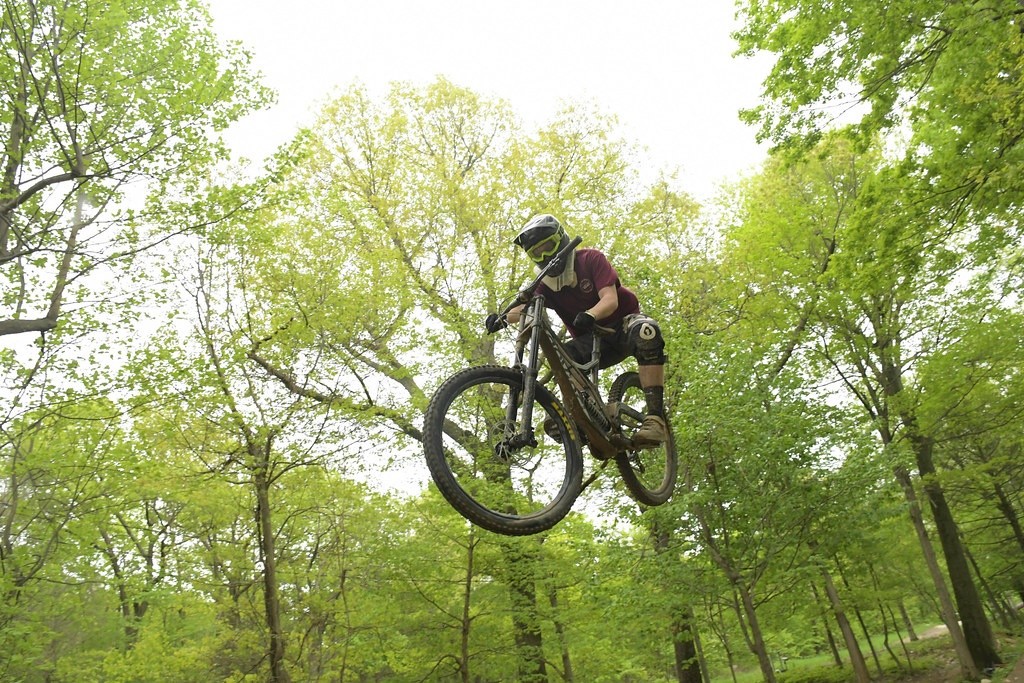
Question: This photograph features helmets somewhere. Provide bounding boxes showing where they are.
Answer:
[514,214,570,277]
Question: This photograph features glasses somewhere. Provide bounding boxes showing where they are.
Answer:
[526,222,564,262]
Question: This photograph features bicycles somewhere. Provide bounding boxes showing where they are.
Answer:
[418,233,681,540]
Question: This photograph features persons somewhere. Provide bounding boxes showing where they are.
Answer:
[487,215,672,445]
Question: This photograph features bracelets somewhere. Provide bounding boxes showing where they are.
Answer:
[586,310,596,321]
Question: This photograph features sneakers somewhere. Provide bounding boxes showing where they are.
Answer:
[544,415,563,444]
[634,415,668,441]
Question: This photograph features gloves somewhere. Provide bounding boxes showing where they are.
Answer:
[486,314,508,333]
[573,312,595,331]
